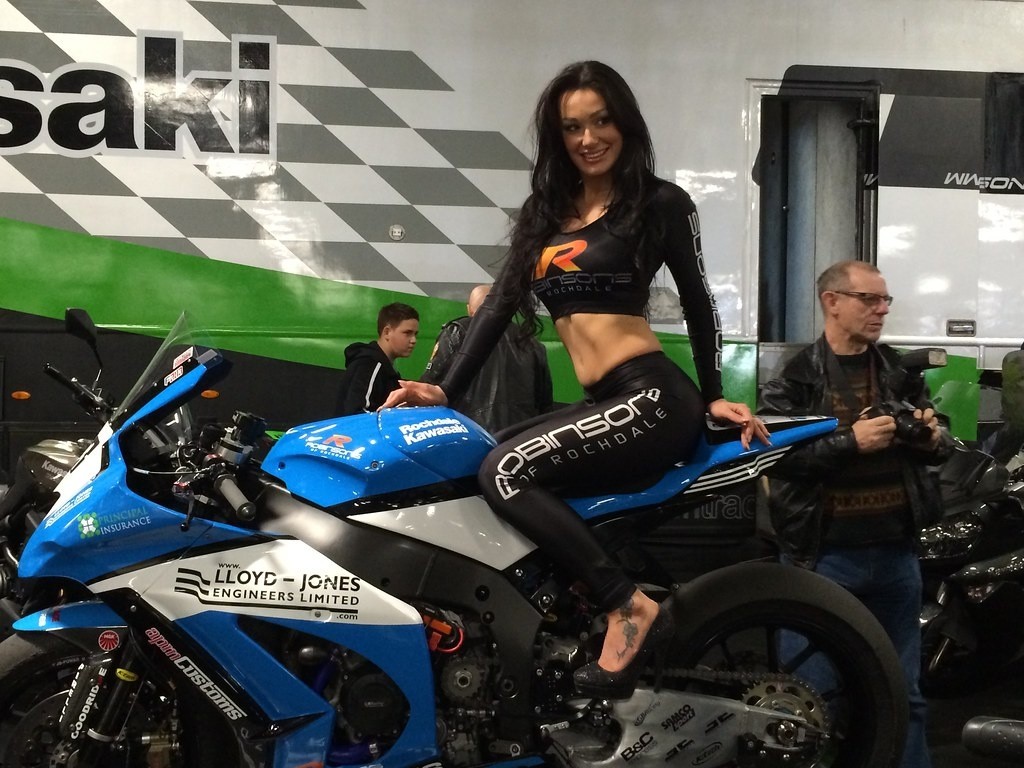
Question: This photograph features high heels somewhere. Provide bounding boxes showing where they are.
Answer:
[573,603,673,698]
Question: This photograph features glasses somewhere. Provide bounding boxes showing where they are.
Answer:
[833,289,893,306]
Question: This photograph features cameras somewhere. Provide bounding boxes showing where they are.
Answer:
[866,399,934,445]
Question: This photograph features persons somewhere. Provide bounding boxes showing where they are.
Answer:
[418,285,555,435]
[377,59,772,698]
[757,262,948,767]
[337,302,421,417]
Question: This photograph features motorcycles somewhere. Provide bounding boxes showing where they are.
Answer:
[917,427,1023,691]
[0,308,909,768]
[962,718,1024,761]
